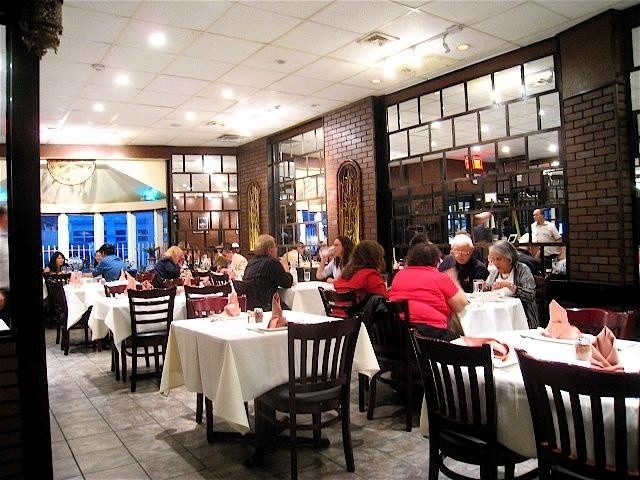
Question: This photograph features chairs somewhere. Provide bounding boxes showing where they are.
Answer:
[565,306,633,341]
[515,346,640,480]
[45,260,519,442]
[408,327,538,480]
[254,316,362,480]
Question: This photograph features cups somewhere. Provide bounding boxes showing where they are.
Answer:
[201,267,205,271]
[472,279,484,294]
[254,308,264,323]
[575,337,592,367]
[61,265,72,273]
[323,248,335,258]
[303,268,310,283]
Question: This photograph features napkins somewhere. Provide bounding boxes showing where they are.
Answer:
[540,299,583,340]
[589,326,625,375]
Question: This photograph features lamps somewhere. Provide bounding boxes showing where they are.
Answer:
[376,29,452,82]
[464,153,484,179]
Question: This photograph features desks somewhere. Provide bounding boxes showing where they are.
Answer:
[428,327,640,476]
[169,310,345,470]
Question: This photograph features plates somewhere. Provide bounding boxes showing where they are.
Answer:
[257,322,288,331]
[491,345,521,368]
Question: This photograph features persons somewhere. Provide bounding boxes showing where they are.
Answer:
[0,205,10,333]
[283,241,305,263]
[153,246,183,288]
[44,251,70,274]
[388,242,467,340]
[531,208,566,269]
[93,244,125,282]
[473,212,499,261]
[206,243,248,281]
[240,235,293,310]
[515,232,540,276]
[316,236,354,283]
[484,239,539,327]
[437,233,490,293]
[332,239,386,317]
